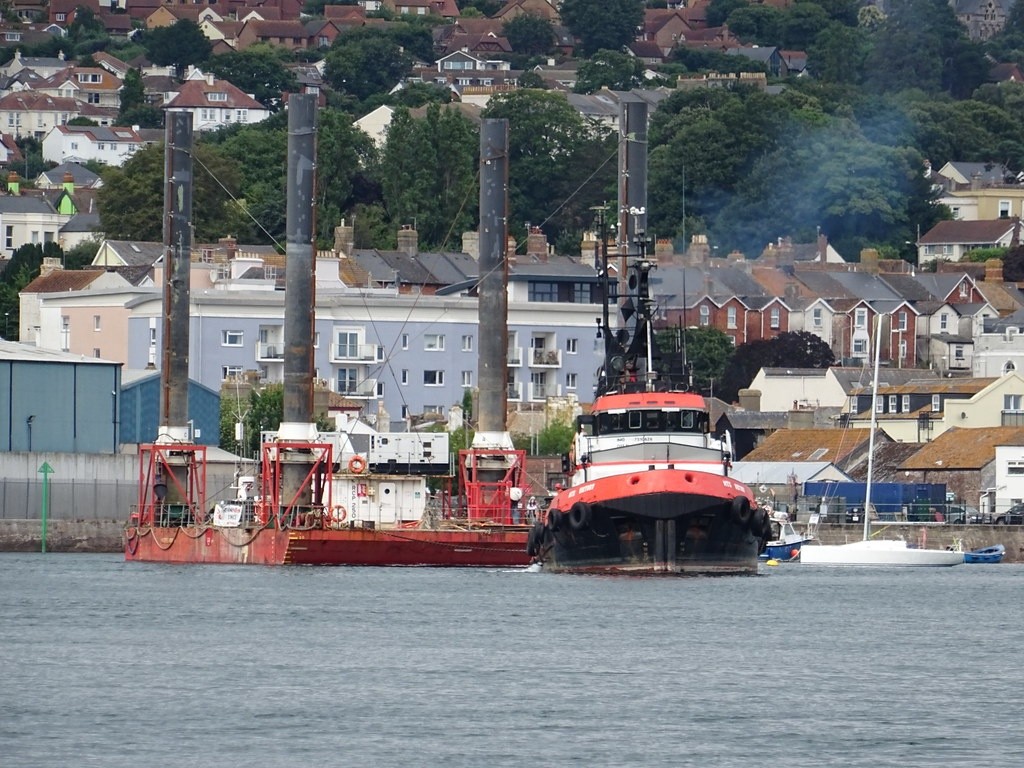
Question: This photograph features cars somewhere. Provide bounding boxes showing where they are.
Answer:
[995,503,1023,530]
[945,491,960,502]
[946,502,982,524]
[843,506,865,524]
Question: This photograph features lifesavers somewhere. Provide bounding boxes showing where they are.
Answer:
[329,506,347,522]
[348,456,366,473]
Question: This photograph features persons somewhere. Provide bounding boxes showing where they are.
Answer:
[554,483,562,494]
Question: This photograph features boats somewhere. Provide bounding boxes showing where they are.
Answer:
[123,89,542,572]
[527,203,780,580]
[758,500,816,564]
[964,542,1007,567]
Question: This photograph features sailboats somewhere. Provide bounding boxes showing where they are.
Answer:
[798,311,967,568]
